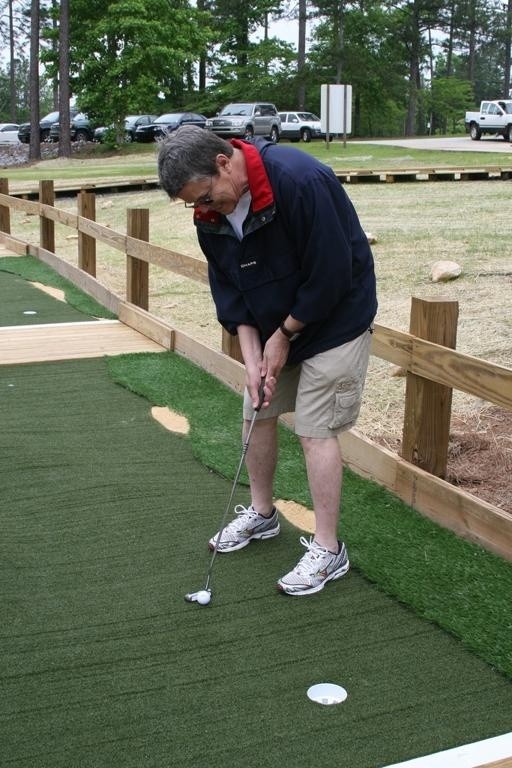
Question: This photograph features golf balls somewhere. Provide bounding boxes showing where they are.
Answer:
[196,590,211,605]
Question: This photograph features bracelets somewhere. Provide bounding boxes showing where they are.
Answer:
[279,319,300,342]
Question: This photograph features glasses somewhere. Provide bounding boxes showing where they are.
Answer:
[185,175,214,209]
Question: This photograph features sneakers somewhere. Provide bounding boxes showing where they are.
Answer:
[208,505,280,553]
[277,536,350,596]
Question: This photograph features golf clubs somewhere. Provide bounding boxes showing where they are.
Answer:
[184,371,266,602]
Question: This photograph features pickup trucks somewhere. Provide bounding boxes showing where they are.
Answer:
[463,99,512,143]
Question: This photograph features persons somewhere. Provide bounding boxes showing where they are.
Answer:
[155,121,377,599]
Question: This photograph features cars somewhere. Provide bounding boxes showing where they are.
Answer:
[0,123,22,147]
[278,111,333,143]
[136,113,208,143]
[93,114,159,144]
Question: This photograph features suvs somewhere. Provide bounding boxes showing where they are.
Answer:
[18,110,78,144]
[49,111,99,143]
[204,101,282,144]
